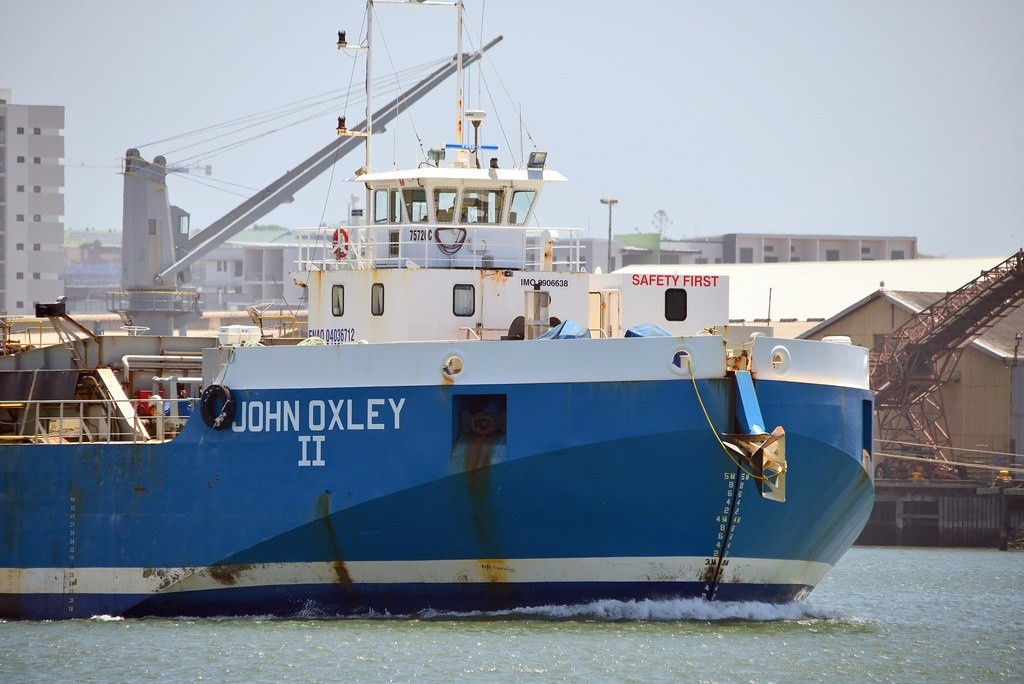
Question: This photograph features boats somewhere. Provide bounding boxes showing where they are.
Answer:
[0,2,878,623]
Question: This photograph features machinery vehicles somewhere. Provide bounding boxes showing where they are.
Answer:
[867,248,1024,480]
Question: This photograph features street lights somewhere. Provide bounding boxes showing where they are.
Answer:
[600,197,619,274]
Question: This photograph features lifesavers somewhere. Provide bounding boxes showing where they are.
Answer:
[333,227,350,258]
[201,382,235,431]
